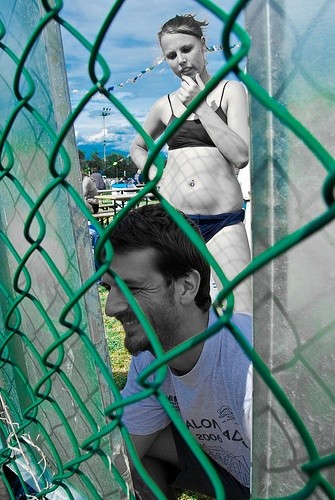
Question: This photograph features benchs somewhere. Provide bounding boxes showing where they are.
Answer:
[93,203,117,225]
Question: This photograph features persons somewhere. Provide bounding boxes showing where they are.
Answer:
[97,203,253,500]
[81,168,142,214]
[131,14,252,315]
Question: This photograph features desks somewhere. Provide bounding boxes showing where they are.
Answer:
[94,184,153,212]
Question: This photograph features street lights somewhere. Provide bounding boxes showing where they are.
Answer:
[102,106,111,164]
